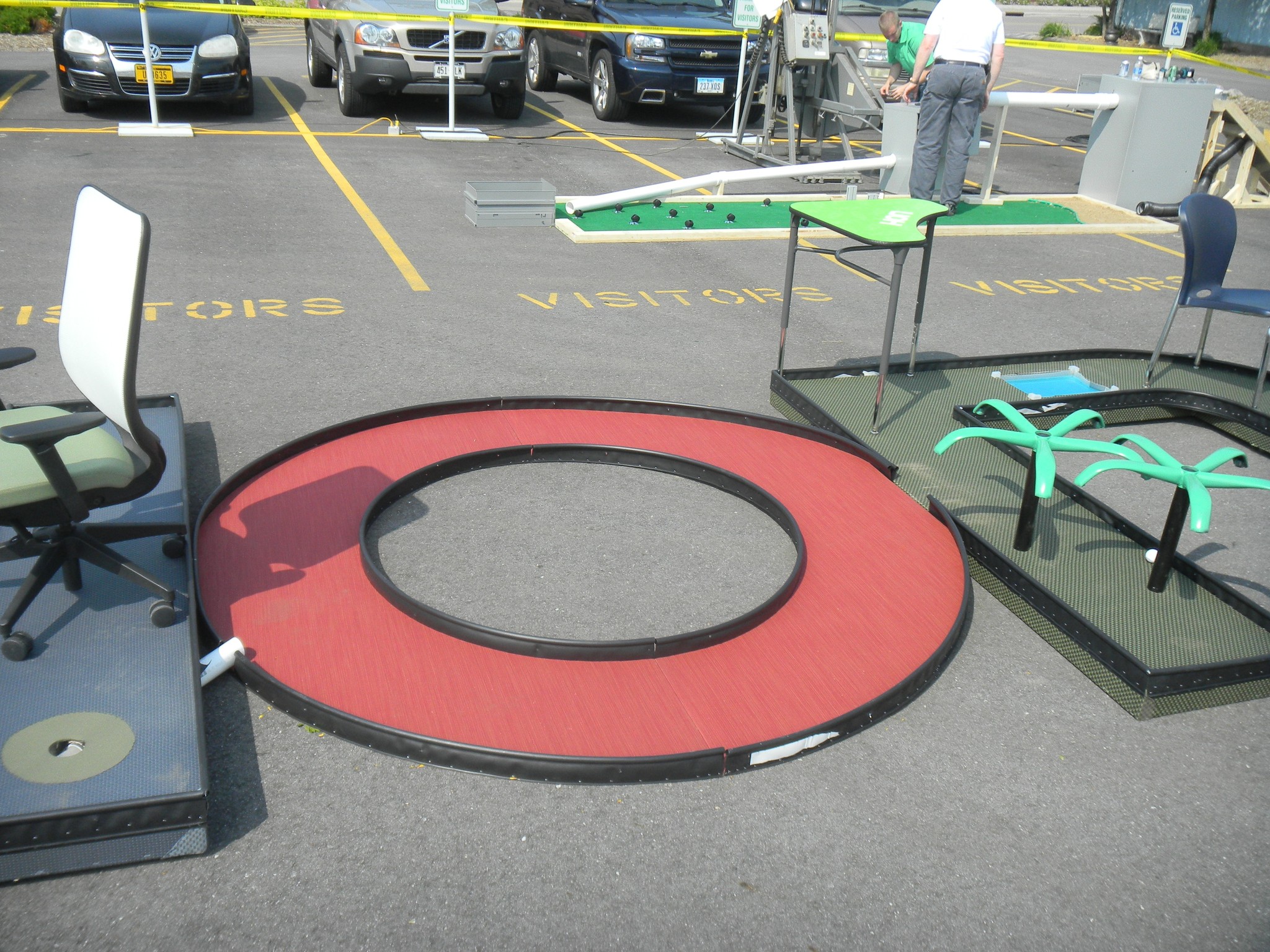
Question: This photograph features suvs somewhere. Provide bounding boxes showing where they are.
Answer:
[791,0,939,102]
[304,0,529,120]
[521,0,781,123]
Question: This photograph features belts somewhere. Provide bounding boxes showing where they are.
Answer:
[935,59,986,68]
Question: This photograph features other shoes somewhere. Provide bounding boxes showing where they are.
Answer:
[944,202,955,216]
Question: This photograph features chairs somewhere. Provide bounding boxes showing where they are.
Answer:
[1143,192,1270,412]
[0,186,188,659]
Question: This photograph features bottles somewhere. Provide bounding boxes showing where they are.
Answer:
[1132,56,1143,81]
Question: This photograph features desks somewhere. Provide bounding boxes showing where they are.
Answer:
[777,198,949,435]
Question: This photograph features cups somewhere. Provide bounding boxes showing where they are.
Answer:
[1119,60,1130,77]
[1186,68,1194,78]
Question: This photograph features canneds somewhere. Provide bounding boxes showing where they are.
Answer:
[1118,60,1130,77]
[1167,65,1178,82]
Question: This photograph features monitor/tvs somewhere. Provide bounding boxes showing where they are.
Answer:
[792,0,829,15]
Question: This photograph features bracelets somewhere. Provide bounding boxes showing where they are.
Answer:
[909,77,918,84]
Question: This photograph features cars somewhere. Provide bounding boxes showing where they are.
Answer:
[52,0,256,115]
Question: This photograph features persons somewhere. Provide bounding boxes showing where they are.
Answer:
[878,11,935,103]
[902,0,1006,217]
